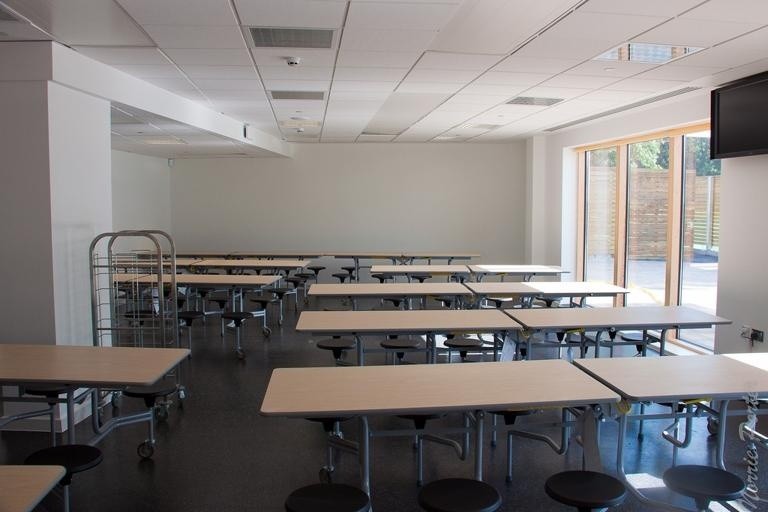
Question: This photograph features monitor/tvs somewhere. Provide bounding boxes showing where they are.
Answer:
[711,70,768,160]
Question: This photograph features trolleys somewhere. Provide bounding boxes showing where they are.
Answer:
[88,225,185,421]
